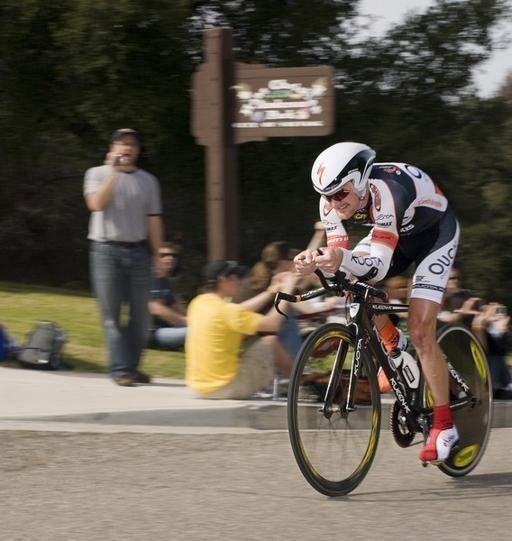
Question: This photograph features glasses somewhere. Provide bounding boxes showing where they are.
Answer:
[324,189,351,202]
[157,253,177,258]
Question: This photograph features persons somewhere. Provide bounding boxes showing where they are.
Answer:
[82,128,168,386]
[149,240,371,406]
[293,142,459,461]
[375,247,512,402]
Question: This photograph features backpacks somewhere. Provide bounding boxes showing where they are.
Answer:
[14,323,65,370]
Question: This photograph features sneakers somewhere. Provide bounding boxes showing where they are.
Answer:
[113,371,150,386]
[377,328,408,393]
[419,424,459,462]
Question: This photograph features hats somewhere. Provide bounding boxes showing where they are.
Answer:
[111,128,138,144]
[205,261,248,285]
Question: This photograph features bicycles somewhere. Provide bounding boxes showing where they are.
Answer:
[273,249,494,498]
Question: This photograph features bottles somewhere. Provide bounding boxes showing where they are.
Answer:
[389,349,421,389]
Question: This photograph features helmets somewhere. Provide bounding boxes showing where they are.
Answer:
[311,142,377,199]
